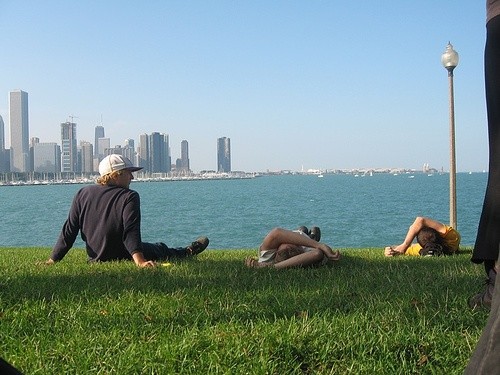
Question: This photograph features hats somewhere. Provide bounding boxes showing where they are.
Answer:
[98,154,144,176]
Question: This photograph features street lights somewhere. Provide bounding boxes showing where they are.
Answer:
[441,41,459,231]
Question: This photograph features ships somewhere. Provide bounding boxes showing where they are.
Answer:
[295,162,322,176]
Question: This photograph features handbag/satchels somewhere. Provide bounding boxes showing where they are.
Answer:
[419,243,441,256]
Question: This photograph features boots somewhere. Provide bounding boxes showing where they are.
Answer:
[470,269,496,306]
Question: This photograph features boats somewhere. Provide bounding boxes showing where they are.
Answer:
[4,170,261,186]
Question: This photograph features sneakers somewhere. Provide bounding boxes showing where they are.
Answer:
[309,227,321,241]
[298,226,308,234]
[186,237,209,256]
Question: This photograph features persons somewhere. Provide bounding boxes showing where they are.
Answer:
[384,217,460,257]
[466,0,500,375]
[45,154,209,268]
[246,225,341,268]
